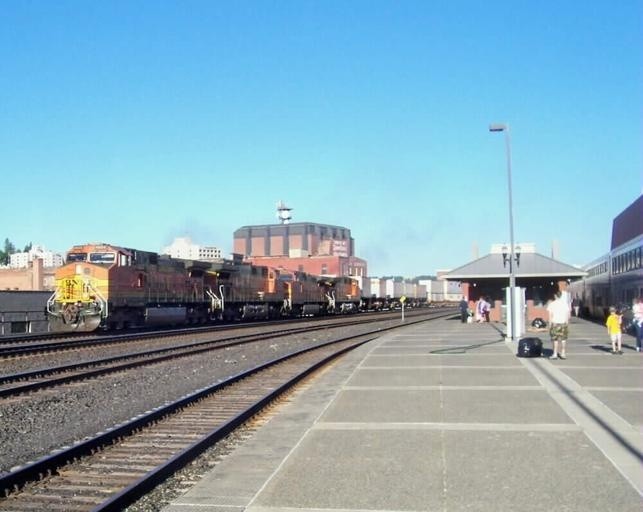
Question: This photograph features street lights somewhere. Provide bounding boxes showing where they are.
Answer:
[489,123,521,343]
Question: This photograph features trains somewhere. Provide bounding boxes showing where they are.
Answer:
[47,243,427,333]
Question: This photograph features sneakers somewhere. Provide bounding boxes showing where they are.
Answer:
[548,353,567,360]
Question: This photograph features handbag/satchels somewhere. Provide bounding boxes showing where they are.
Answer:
[517,336,544,358]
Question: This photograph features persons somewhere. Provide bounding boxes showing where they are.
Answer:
[624,296,643,353]
[475,296,486,324]
[483,296,490,322]
[545,281,573,360]
[459,296,468,323]
[605,305,624,354]
[573,296,582,317]
